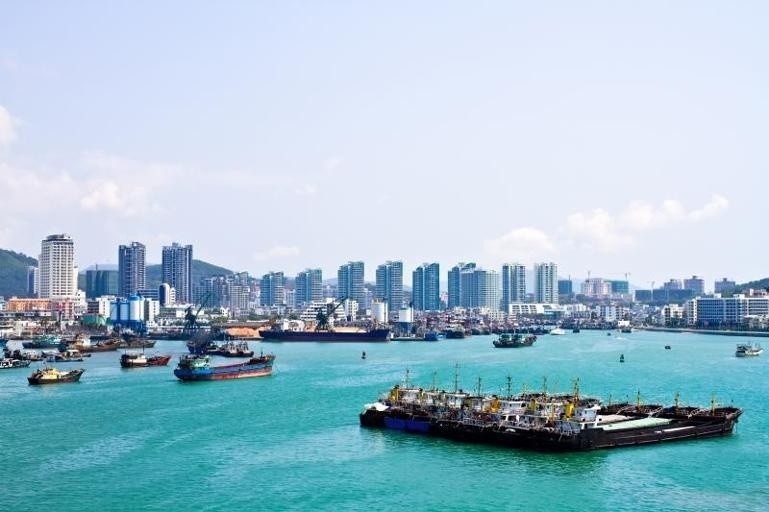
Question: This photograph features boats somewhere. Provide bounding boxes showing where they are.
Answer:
[360,351,366,359]
[664,345,671,350]
[256,295,391,343]
[735,343,746,357]
[619,353,625,362]
[744,348,763,356]
[422,321,633,349]
[0,327,280,386]
[145,289,234,340]
